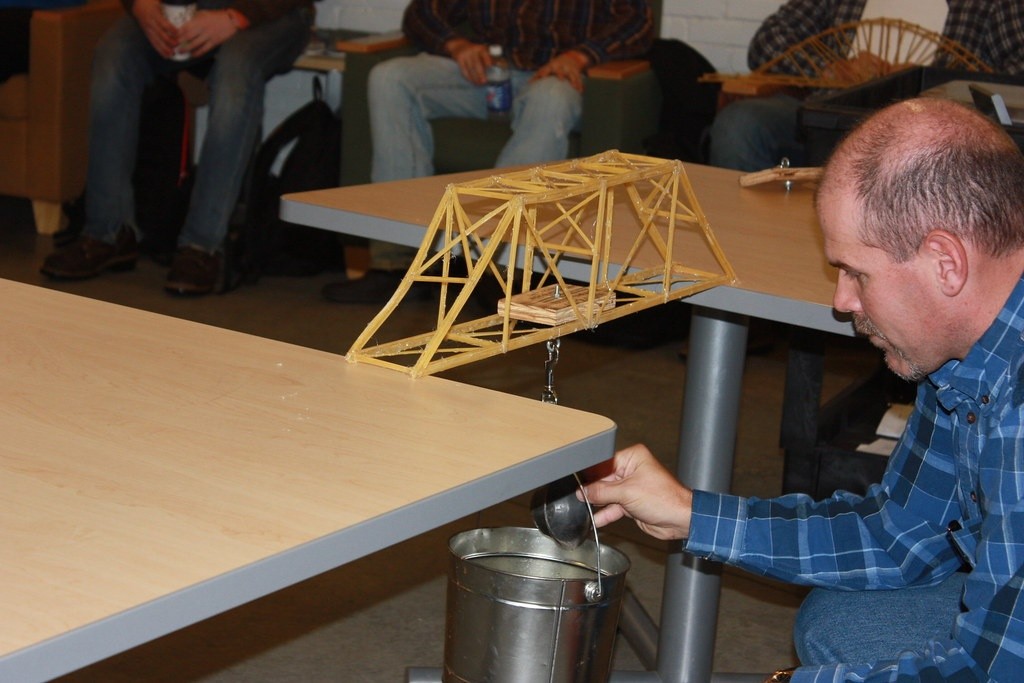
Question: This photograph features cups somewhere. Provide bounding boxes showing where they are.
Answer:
[160,2,196,61]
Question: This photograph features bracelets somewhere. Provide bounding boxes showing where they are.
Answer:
[225,9,242,34]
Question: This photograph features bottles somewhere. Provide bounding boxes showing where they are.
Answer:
[483,43,514,127]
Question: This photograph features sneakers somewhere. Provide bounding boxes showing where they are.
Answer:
[39,223,137,278]
[163,244,219,293]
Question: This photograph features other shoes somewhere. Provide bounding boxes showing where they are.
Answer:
[332,266,433,302]
[676,319,776,360]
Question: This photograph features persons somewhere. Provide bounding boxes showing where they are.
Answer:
[577,0,1024,682]
[321,2,655,317]
[39,1,317,299]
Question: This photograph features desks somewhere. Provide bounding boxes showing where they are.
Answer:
[0,278,618,683]
[276,158,852,683]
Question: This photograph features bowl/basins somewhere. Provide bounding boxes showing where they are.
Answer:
[530,471,594,552]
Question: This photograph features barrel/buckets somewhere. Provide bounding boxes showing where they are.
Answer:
[442,473,630,683]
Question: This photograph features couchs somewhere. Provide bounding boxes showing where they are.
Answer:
[0,0,132,236]
[337,32,652,301]
